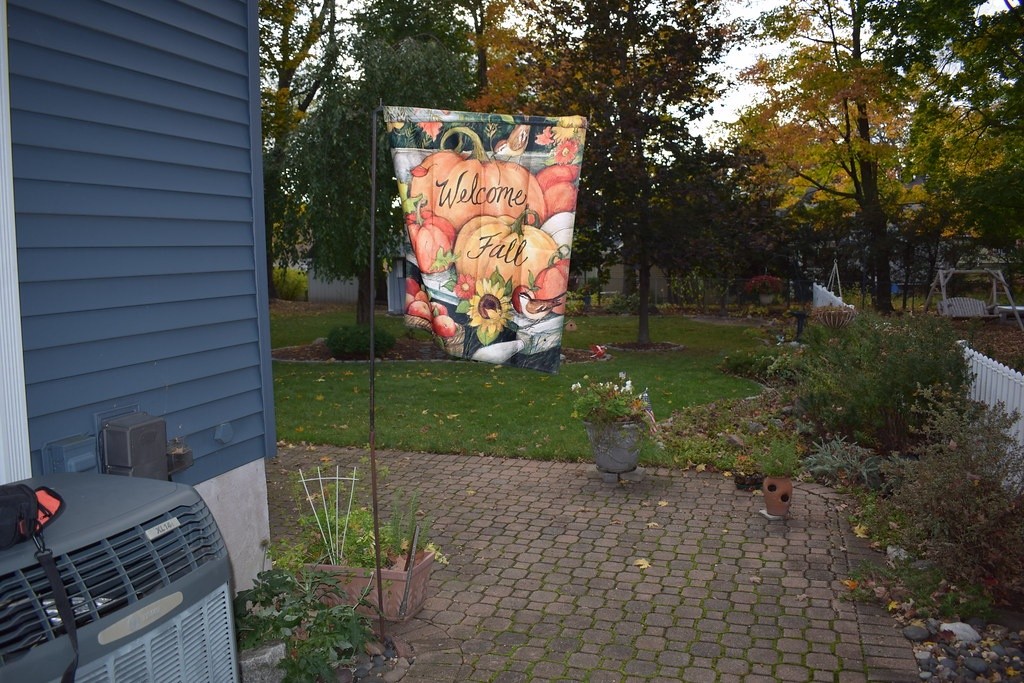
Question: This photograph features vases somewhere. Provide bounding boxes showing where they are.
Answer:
[580,418,646,474]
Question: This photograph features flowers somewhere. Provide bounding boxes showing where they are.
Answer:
[571,371,666,467]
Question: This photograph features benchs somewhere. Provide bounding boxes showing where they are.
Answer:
[937,296,1002,321]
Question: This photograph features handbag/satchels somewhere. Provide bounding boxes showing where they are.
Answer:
[0,482,67,550]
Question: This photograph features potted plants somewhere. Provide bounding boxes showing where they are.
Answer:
[758,433,801,517]
[230,444,450,683]
